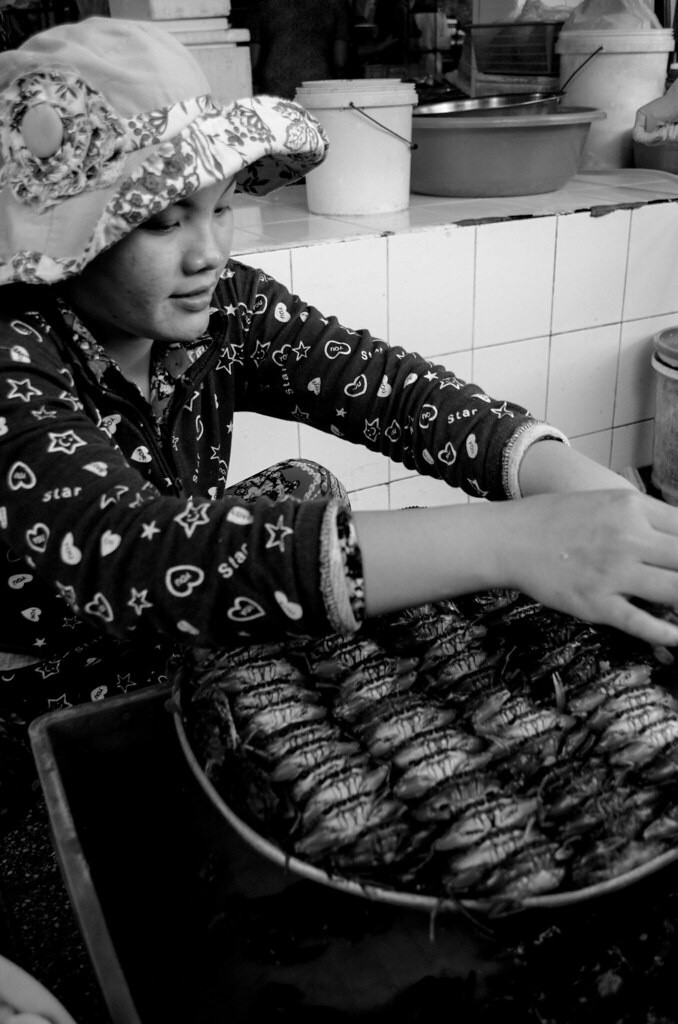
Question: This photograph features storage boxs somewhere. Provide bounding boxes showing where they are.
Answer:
[27,664,678,1024]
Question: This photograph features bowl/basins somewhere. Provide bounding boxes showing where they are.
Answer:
[413,92,567,117]
[171,665,677,911]
[410,107,607,199]
[655,327,678,367]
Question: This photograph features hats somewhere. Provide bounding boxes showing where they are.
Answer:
[0,16,331,286]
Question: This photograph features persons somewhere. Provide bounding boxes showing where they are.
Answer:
[632,78,678,146]
[0,15,678,1024]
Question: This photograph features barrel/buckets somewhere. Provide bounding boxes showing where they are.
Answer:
[651,352,678,496]
[554,29,676,171]
[294,77,419,216]
